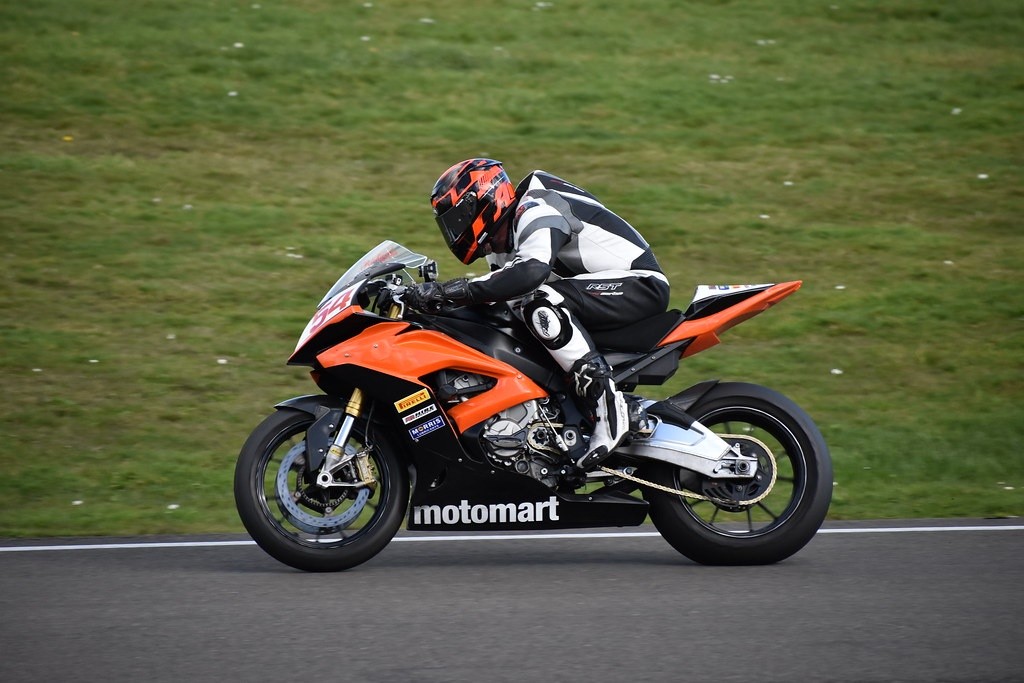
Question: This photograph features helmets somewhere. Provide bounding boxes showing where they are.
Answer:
[430,157,517,265]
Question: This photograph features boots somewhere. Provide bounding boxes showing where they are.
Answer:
[577,349,648,470]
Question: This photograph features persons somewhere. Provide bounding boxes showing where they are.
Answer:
[403,157,670,470]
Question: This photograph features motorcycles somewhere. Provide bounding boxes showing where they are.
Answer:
[231,237,833,575]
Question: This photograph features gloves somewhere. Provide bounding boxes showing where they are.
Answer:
[411,276,474,314]
